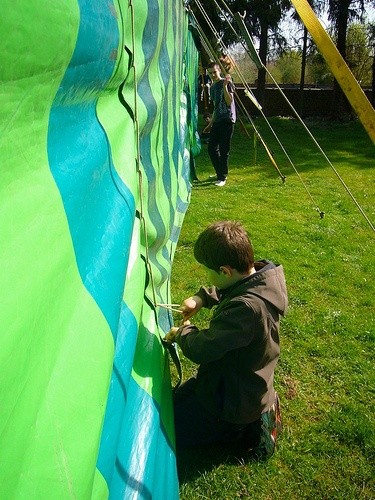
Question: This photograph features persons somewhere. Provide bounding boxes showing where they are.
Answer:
[198,61,237,188]
[163,219,289,461]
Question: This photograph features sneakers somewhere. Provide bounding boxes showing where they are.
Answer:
[248,391,282,461]
[215,179,226,186]
[209,173,227,180]
